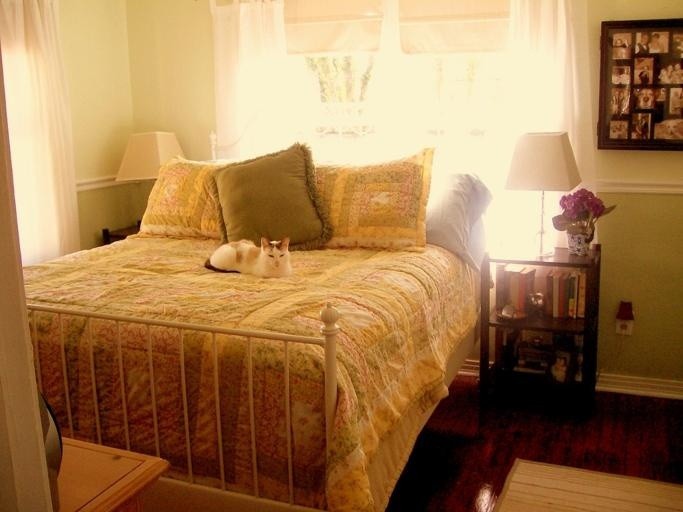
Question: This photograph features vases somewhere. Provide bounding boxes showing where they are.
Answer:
[565,228,591,258]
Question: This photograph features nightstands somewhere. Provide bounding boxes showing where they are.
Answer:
[99,218,142,245]
[476,238,603,423]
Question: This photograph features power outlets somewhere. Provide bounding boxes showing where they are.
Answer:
[612,314,635,337]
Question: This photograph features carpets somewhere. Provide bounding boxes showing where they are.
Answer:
[490,453,683,511]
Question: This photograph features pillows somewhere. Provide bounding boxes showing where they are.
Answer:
[304,147,432,254]
[414,167,493,272]
[205,140,331,255]
[134,154,238,242]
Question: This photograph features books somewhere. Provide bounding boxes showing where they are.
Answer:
[495,264,585,320]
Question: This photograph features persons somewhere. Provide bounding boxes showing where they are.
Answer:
[635,32,650,55]
[659,63,683,84]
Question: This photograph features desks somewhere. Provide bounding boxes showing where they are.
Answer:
[47,432,171,511]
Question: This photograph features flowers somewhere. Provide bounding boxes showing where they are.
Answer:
[551,187,618,236]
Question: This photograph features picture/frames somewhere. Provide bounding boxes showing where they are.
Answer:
[593,16,683,154]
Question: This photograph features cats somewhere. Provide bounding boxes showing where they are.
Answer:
[203,236,293,279]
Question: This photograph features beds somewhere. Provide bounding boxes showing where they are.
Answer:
[17,209,493,512]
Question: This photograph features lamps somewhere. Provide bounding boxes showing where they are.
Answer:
[496,126,584,259]
[112,130,187,230]
